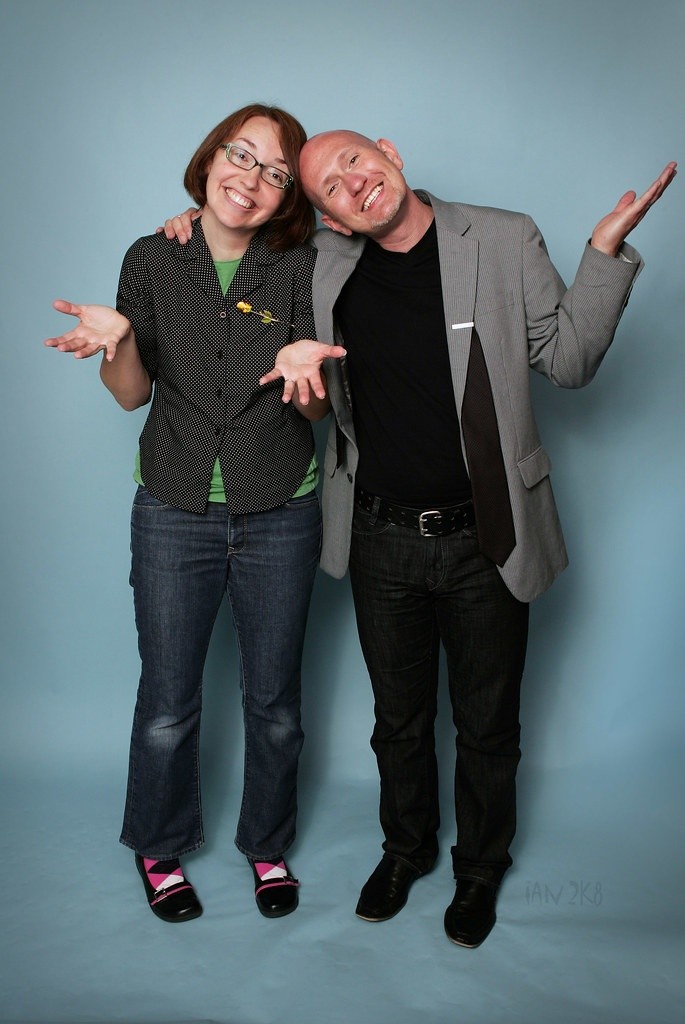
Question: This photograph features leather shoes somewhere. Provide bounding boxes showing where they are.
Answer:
[444,882,498,949]
[135,851,203,923]
[355,853,416,923]
[247,853,301,918]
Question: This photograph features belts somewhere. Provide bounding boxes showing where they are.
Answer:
[358,488,476,535]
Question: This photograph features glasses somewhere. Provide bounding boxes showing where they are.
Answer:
[220,141,294,190]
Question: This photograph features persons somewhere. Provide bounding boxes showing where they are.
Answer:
[44,102,347,923]
[156,129,677,949]
